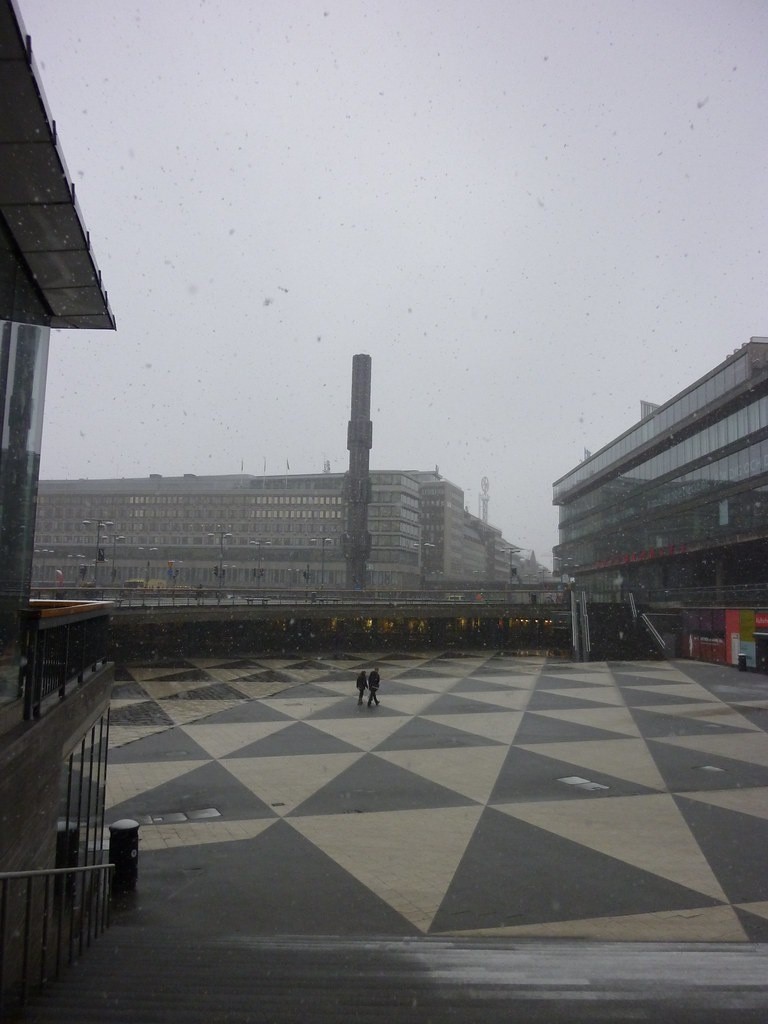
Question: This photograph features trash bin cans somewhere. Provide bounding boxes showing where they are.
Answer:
[737,653,747,672]
[108,819,140,894]
[54,822,80,894]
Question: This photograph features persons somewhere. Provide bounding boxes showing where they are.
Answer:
[356,670,368,706]
[367,667,380,707]
[195,584,203,606]
